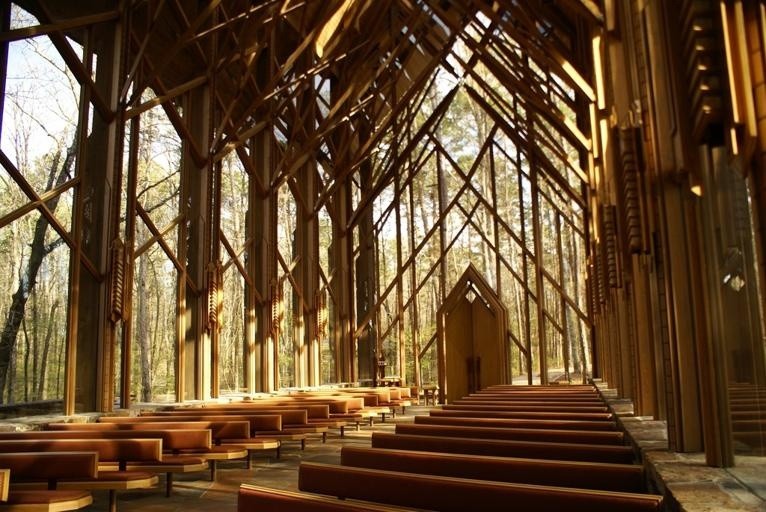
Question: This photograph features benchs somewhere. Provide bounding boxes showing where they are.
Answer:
[0,385,665,512]
